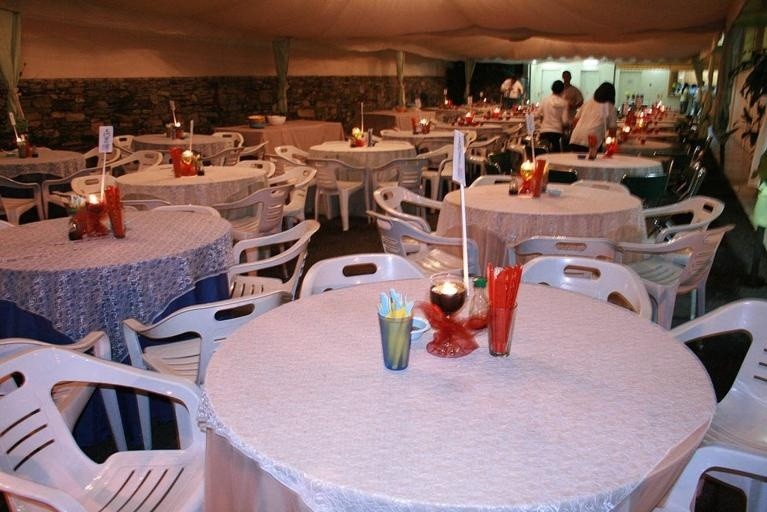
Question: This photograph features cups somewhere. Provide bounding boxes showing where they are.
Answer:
[520,165,535,181]
[485,298,518,357]
[377,311,413,370]
[428,272,467,316]
[170,158,183,179]
[108,207,128,238]
[181,154,193,165]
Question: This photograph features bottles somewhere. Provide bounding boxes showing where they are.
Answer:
[469,276,488,328]
[531,157,545,198]
[67,208,82,239]
[195,158,206,176]
[509,168,518,195]
[30,145,38,157]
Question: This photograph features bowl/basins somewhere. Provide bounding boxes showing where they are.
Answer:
[268,115,285,125]
[548,189,561,197]
[247,116,265,128]
[409,316,429,339]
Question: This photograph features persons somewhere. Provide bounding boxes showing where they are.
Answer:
[500,73,524,110]
[561,70,584,120]
[537,80,573,153]
[568,81,618,154]
[677,79,717,115]
